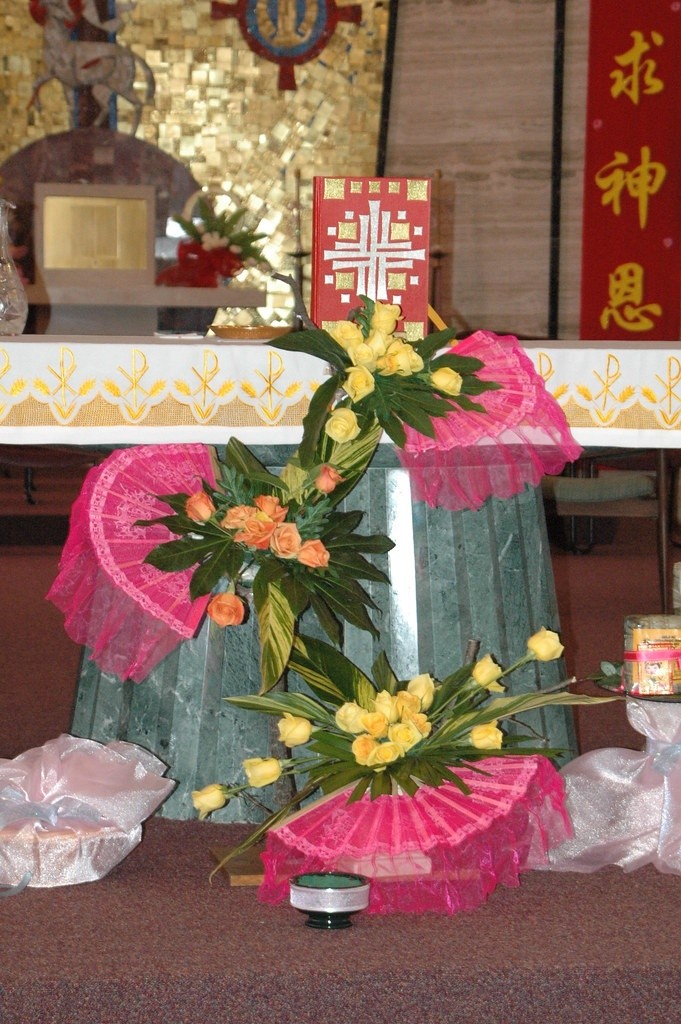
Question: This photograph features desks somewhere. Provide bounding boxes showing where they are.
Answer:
[1,333,679,617]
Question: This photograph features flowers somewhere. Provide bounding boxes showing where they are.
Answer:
[150,292,504,697]
[174,625,627,884]
